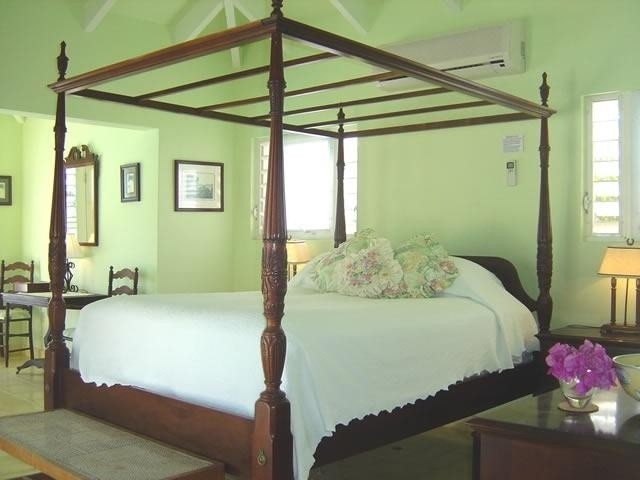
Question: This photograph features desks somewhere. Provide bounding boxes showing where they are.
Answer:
[0,292,108,375]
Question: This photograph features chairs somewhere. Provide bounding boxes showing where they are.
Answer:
[62,266,138,342]
[0,260,34,368]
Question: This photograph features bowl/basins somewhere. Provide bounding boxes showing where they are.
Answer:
[612,353,640,402]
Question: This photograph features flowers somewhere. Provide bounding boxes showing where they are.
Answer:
[545,340,617,395]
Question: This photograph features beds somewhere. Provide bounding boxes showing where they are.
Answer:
[43,0,557,480]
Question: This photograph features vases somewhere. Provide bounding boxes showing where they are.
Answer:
[557,370,599,413]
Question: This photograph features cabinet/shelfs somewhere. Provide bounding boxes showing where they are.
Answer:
[464,381,640,480]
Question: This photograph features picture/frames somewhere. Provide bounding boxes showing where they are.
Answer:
[0,175,12,205]
[120,162,140,202]
[174,160,224,212]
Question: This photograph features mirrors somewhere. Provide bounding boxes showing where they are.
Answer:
[64,145,98,247]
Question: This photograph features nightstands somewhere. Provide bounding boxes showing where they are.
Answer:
[535,326,640,394]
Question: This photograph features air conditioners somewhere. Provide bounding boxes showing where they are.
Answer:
[373,19,526,92]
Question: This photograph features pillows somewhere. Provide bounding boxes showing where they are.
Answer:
[288,227,505,313]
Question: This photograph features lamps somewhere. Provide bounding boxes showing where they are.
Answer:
[597,238,640,336]
[62,233,79,294]
